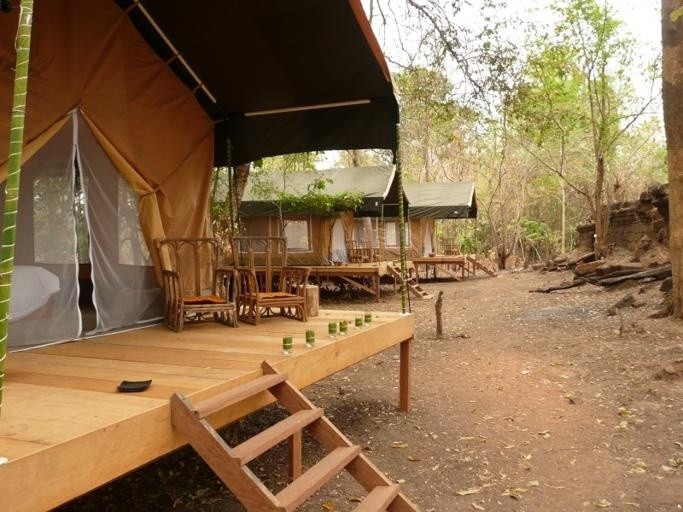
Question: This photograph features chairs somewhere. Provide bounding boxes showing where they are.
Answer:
[345,239,369,264]
[360,240,384,262]
[149,235,241,335]
[227,234,313,329]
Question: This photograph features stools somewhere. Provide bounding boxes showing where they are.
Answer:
[296,283,320,318]
